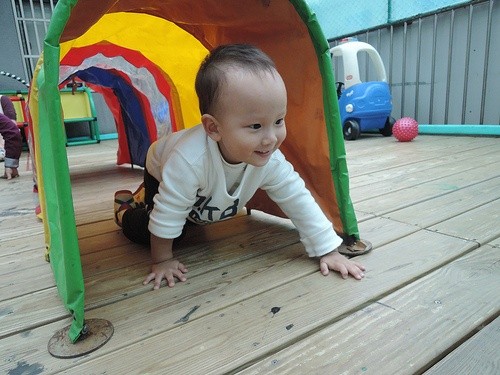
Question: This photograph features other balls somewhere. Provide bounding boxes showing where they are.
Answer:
[392,117,418,142]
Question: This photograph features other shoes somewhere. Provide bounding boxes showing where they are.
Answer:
[114,190,136,226]
[133,182,145,203]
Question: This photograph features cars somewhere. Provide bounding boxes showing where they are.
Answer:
[330,34,398,142]
